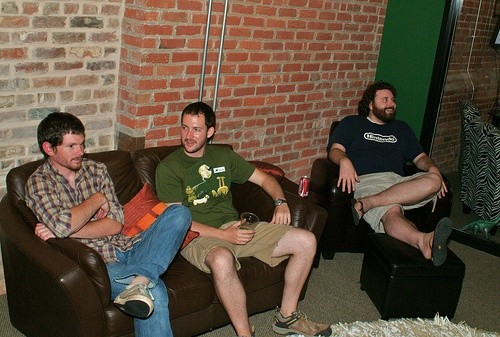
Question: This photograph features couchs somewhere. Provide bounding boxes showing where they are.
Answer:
[0,145,328,337]
[309,121,451,260]
[458,99,500,237]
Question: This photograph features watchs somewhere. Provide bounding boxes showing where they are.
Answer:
[273,199,287,207]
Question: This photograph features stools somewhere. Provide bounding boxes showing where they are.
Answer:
[360,232,466,321]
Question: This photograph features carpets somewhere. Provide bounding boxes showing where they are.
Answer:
[287,312,500,337]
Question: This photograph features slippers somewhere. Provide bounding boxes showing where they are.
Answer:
[352,199,364,226]
[431,217,452,266]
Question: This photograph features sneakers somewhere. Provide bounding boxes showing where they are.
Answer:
[113,282,154,318]
[273,306,332,337]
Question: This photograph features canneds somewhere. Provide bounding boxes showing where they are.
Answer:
[298,176,310,197]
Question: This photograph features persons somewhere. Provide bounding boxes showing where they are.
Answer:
[156,101,332,337]
[328,82,453,266]
[25,112,193,337]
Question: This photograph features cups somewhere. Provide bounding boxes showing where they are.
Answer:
[240,212,259,230]
